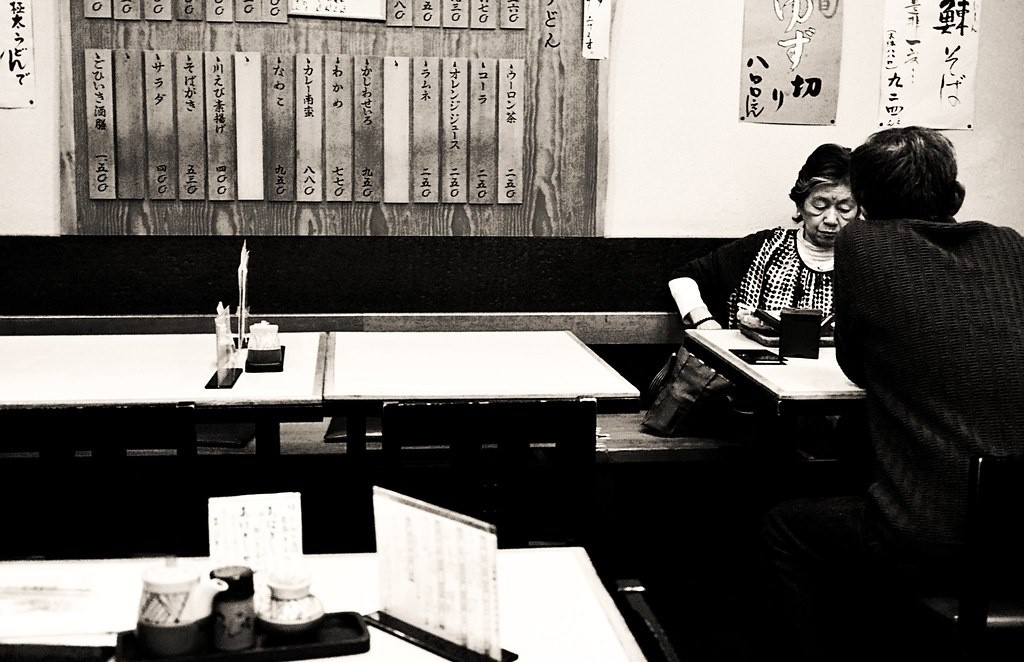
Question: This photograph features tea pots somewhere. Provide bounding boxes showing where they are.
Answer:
[137,565,230,655]
[247,321,281,365]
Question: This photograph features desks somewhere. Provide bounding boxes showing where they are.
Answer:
[685,328,868,419]
[0,547,650,662]
[325,330,643,458]
[0,332,324,455]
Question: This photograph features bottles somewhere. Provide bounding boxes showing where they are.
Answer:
[210,567,255,650]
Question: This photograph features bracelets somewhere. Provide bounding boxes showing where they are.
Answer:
[694,316,714,327]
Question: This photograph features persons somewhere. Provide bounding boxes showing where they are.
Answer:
[739,124,1024,661]
[666,143,865,516]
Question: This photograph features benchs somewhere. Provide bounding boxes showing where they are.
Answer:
[0,311,753,456]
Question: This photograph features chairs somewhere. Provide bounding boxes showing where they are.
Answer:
[903,455,1024,662]
[382,396,601,511]
[0,400,198,456]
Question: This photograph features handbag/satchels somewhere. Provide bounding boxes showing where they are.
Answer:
[641,345,739,436]
